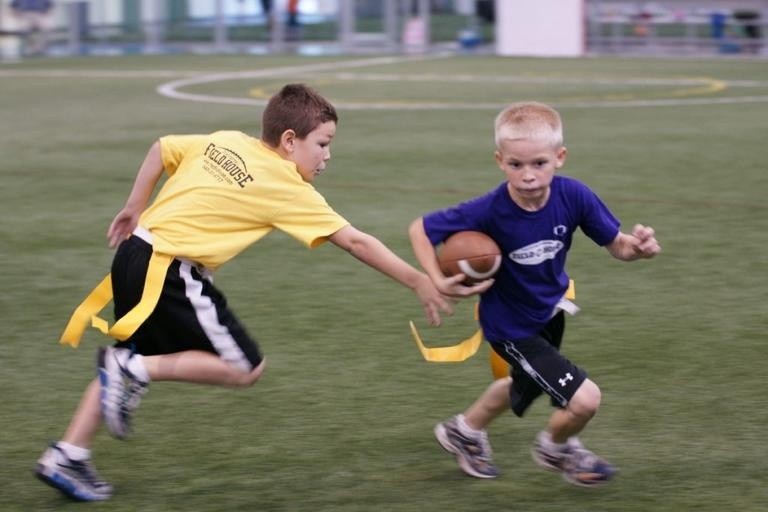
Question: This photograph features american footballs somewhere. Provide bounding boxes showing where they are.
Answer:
[441,233,502,286]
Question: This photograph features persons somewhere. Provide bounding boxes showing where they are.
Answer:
[404,101,662,491]
[260,0,300,43]
[32,84,463,503]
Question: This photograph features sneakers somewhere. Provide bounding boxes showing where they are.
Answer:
[96,345,149,441]
[32,442,113,500]
[531,430,619,488]
[434,413,500,479]
